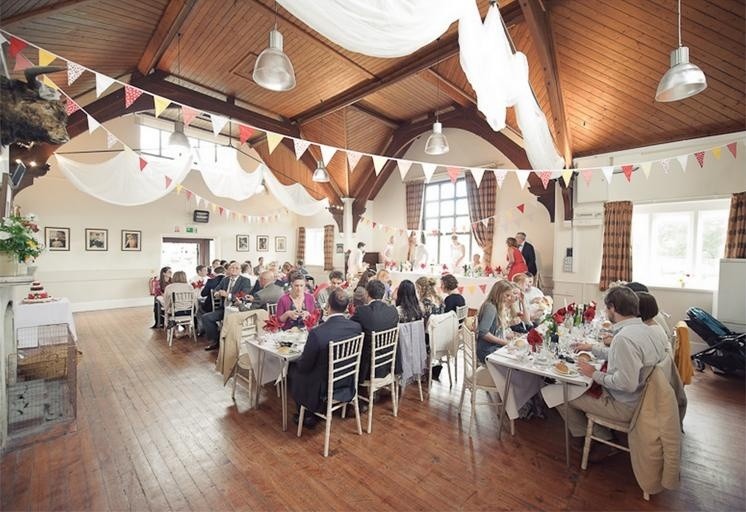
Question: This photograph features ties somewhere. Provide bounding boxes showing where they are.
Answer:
[229,278,235,292]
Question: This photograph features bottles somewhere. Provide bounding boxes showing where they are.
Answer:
[298,328,306,343]
[545,304,587,356]
[399,261,402,272]
[304,328,309,338]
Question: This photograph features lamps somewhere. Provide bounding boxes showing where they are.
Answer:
[313,100,330,183]
[425,62,449,155]
[253,0,296,92]
[194,210,211,222]
[655,0,707,102]
[168,34,190,150]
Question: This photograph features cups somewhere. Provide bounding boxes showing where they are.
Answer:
[429,264,434,273]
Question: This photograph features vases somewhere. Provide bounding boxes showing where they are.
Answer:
[0,251,24,276]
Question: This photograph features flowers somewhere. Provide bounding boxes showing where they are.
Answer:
[0,215,47,264]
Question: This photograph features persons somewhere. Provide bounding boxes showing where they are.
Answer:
[447,233,466,268]
[383,234,396,263]
[514,231,538,276]
[345,241,369,275]
[475,270,677,420]
[124,233,138,248]
[502,237,529,280]
[277,272,466,428]
[553,285,666,464]
[409,234,430,270]
[470,253,483,270]
[49,231,67,248]
[150,256,309,350]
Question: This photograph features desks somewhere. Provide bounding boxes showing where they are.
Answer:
[14,297,77,349]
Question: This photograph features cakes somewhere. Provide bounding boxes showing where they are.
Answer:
[23,281,53,303]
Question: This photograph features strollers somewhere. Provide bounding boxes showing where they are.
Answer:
[685,307,746,377]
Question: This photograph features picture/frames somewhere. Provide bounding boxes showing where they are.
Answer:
[85,228,108,251]
[256,235,269,252]
[121,230,141,251]
[44,227,70,251]
[236,235,249,252]
[275,236,287,252]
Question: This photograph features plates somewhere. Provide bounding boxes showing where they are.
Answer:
[551,358,581,377]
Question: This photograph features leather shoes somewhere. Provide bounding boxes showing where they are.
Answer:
[587,443,620,461]
[204,343,218,351]
[293,415,314,428]
